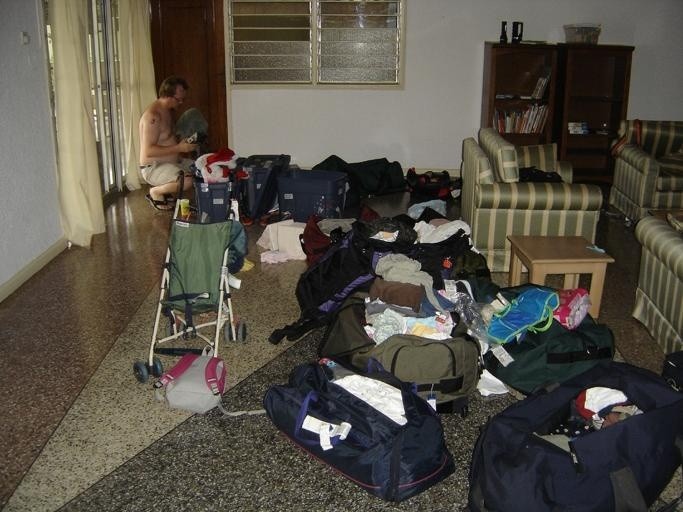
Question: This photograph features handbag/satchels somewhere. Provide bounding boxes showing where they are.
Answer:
[190,172,235,224]
[277,168,351,222]
[240,153,293,221]
[258,351,457,503]
[288,210,618,414]
[463,359,683,511]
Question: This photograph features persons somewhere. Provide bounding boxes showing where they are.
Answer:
[139,77,200,212]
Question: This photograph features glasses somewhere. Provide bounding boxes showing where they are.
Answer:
[171,95,185,105]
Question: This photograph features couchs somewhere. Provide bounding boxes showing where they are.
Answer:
[608,119,683,228]
[634,213,683,357]
[460,128,603,274]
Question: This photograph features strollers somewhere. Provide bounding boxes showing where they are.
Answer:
[132,171,246,384]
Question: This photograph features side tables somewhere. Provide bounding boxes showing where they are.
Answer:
[505,235,616,319]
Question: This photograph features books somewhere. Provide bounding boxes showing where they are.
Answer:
[492,101,549,135]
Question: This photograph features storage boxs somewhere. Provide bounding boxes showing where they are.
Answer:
[194,165,351,224]
[564,24,601,45]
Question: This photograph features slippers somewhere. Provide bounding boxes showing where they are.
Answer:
[145,192,177,212]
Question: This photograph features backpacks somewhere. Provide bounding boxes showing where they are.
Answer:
[152,344,228,417]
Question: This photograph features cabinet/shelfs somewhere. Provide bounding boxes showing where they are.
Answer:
[482,41,634,188]
